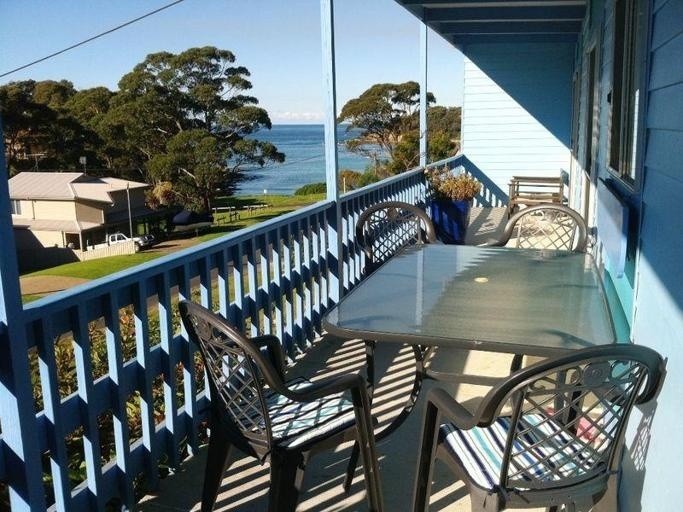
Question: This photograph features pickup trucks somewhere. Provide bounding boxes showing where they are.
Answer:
[87,230,156,254]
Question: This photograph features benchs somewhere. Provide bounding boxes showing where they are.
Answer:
[506,176,565,218]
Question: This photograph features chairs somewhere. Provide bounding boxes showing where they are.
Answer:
[175,298,666,511]
[354,202,590,276]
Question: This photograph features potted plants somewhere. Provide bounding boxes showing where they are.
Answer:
[423,166,483,242]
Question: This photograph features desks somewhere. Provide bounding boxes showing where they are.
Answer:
[321,243,617,493]
